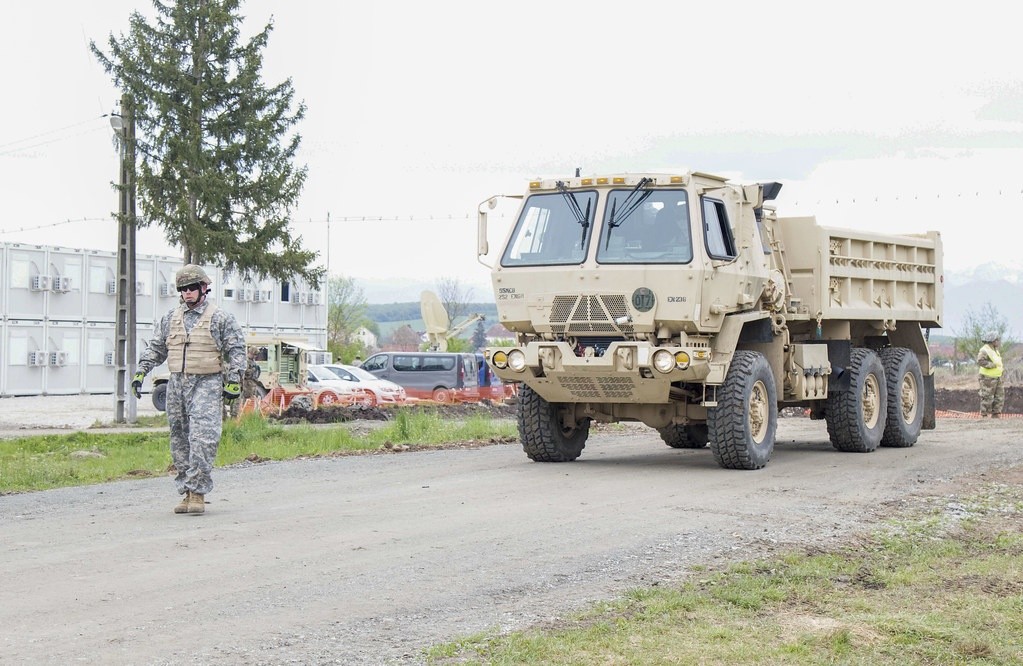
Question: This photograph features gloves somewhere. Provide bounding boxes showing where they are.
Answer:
[222,380,241,407]
[131,371,144,399]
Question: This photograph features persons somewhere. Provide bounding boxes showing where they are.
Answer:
[132,264,247,513]
[242,345,260,400]
[978,331,1004,419]
[653,206,686,246]
[221,361,239,421]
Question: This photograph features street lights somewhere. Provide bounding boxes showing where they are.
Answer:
[109,116,137,424]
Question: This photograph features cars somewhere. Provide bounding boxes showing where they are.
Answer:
[319,364,406,408]
[304,364,364,410]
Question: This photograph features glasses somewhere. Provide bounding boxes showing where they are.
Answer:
[180,281,206,293]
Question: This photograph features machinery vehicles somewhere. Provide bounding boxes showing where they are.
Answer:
[152,334,317,421]
[477,167,943,472]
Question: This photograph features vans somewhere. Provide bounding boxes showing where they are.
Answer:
[459,353,491,389]
[358,352,464,403]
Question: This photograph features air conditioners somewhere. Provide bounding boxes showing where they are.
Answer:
[55,276,71,291]
[240,289,252,301]
[110,279,117,293]
[308,293,320,304]
[136,282,145,295]
[255,291,267,300]
[295,292,308,302]
[34,274,52,290]
[161,282,177,296]
[50,352,67,366]
[29,350,48,367]
[106,351,115,365]
[320,353,332,364]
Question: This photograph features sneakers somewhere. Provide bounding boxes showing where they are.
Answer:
[187,491,204,512]
[174,492,189,513]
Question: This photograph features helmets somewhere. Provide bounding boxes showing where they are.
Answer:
[982,332,999,343]
[175,263,211,292]
